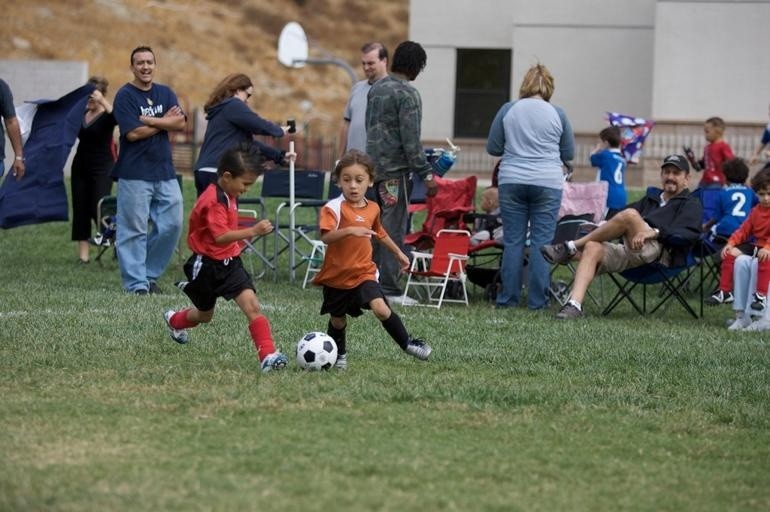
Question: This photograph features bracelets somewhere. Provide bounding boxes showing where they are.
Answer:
[335,160,340,167]
[654,227,660,240]
[15,154,25,161]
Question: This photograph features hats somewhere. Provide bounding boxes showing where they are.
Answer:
[661,154,689,172]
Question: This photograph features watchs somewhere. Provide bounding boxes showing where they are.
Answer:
[422,174,433,182]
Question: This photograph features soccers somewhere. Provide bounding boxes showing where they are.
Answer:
[297,332,338,371]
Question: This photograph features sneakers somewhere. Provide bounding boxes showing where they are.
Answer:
[751,293,766,311]
[334,352,346,370]
[556,301,584,320]
[704,290,735,307]
[163,310,189,344]
[135,282,162,295]
[260,352,289,377]
[728,312,770,331]
[539,241,576,265]
[405,337,432,360]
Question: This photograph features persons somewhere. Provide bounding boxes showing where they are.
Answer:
[589,128,628,218]
[72,76,119,264]
[705,167,769,311]
[364,42,437,305]
[160,147,289,375]
[192,75,297,210]
[314,152,433,373]
[486,64,576,310]
[329,41,389,181]
[0,78,27,186]
[476,186,503,231]
[748,121,769,166]
[702,157,760,239]
[540,152,705,320]
[113,46,187,296]
[683,116,736,187]
